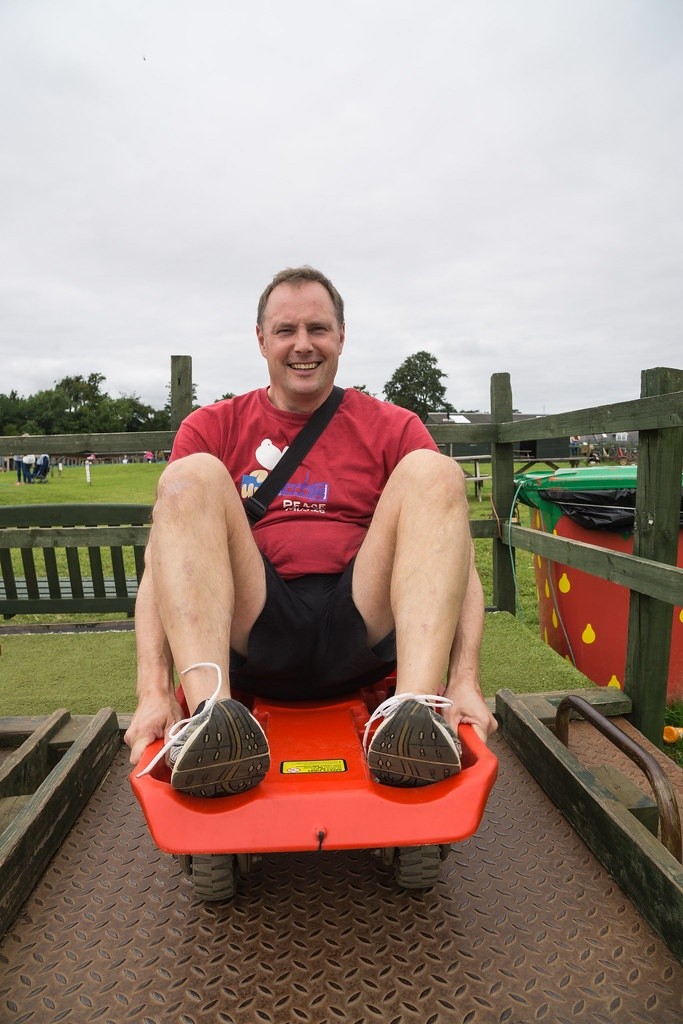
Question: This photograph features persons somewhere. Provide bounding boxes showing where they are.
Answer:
[570,436,581,468]
[123,266,499,796]
[146,450,153,462]
[14,454,35,485]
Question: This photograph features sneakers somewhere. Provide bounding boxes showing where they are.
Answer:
[136,662,270,799]
[362,695,460,789]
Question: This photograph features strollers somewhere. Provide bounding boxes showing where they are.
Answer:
[30,456,48,484]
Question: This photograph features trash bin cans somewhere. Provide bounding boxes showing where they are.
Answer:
[514,465,683,708]
